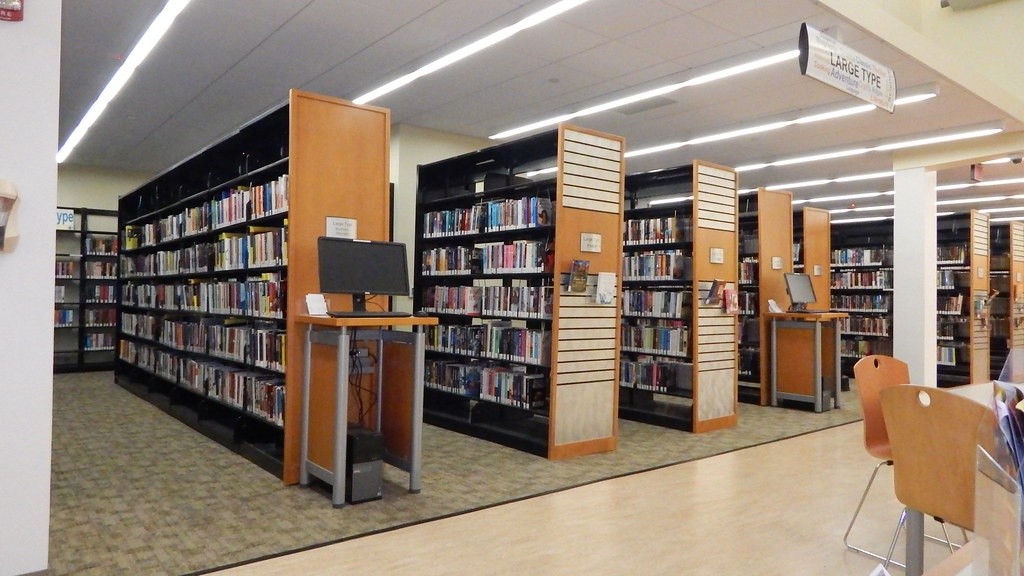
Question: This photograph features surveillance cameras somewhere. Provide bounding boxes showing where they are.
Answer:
[1010,154,1022,164]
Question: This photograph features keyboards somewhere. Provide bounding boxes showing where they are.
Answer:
[325,311,412,318]
[786,310,827,313]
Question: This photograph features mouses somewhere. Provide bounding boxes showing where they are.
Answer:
[829,310,839,313]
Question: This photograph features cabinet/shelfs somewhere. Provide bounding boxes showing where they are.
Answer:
[989,221,1024,382]
[618,159,739,434]
[831,209,990,390]
[412,122,626,462]
[53,206,118,375]
[739,189,794,406]
[793,206,831,313]
[117,89,391,486]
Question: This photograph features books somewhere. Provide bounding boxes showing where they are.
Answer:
[85,308,116,327]
[417,197,556,414]
[85,261,117,279]
[937,246,1008,367]
[619,216,759,394]
[56,260,74,278]
[86,237,117,256]
[55,309,73,327]
[831,248,892,359]
[794,242,804,265]
[86,284,116,303]
[117,173,290,427]
[83,332,116,351]
[55,285,65,303]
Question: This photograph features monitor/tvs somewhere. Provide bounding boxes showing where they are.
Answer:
[318,236,410,312]
[784,272,817,310]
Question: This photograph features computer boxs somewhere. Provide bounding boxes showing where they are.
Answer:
[822,375,830,413]
[316,422,383,503]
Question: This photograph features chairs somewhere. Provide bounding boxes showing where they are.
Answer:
[875,384,999,576]
[844,354,969,568]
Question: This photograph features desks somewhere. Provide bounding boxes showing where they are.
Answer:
[919,379,1024,411]
[294,313,438,508]
[764,313,850,414]
